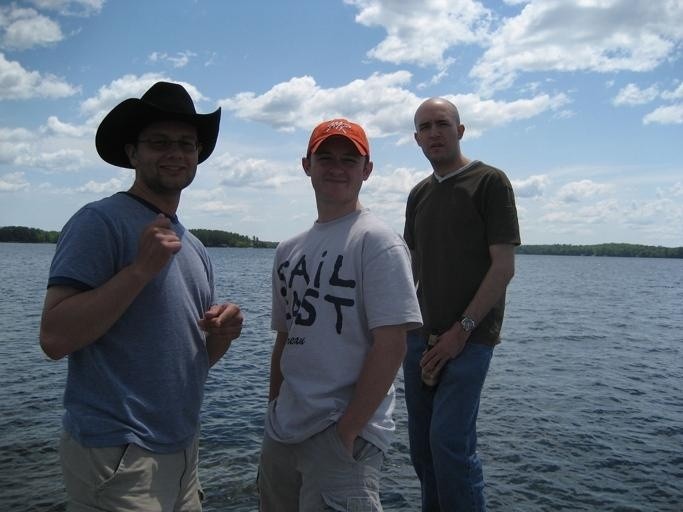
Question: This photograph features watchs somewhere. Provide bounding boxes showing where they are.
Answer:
[456,316,477,333]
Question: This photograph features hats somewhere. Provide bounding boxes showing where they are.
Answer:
[95,81,221,168]
[307,119,370,161]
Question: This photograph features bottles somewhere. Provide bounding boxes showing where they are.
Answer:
[420,327,441,391]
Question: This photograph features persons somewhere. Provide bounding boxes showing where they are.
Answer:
[40,82,244,511]
[256,118,425,512]
[404,97,521,512]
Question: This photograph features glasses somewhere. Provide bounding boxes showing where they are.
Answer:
[139,133,199,153]
